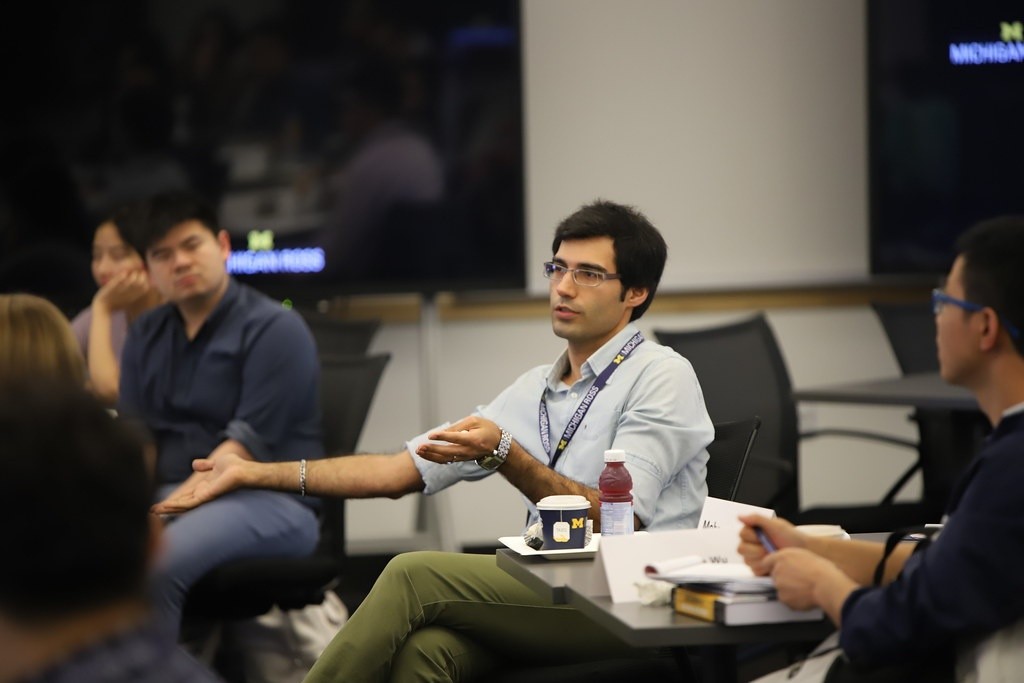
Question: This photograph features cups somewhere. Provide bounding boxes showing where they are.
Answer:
[536,494,592,549]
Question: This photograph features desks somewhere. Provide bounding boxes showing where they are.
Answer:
[497,529,921,683]
[795,371,983,522]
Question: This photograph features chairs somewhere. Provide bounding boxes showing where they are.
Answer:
[871,302,985,524]
[655,310,930,534]
[474,416,760,683]
[152,312,393,682]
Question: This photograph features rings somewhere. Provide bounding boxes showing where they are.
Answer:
[451,456,456,464]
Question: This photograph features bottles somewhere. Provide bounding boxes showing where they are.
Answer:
[597,449,637,538]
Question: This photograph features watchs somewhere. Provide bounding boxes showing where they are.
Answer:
[475,427,513,471]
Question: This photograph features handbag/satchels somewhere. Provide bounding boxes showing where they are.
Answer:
[252,591,347,683]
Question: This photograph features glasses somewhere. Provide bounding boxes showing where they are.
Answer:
[544,262,622,287]
[931,288,1020,337]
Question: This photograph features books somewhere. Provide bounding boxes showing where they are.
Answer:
[671,577,824,626]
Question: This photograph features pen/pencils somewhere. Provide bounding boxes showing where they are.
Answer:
[753,526,777,553]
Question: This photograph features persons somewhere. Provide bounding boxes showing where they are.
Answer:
[0,293,221,683]
[68,207,162,405]
[162,197,715,683]
[118,194,329,647]
[737,214,1023,683]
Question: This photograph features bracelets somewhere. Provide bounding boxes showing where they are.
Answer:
[300,459,306,496]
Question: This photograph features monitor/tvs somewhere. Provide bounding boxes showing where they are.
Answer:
[0,0,526,297]
[865,0,1024,277]
